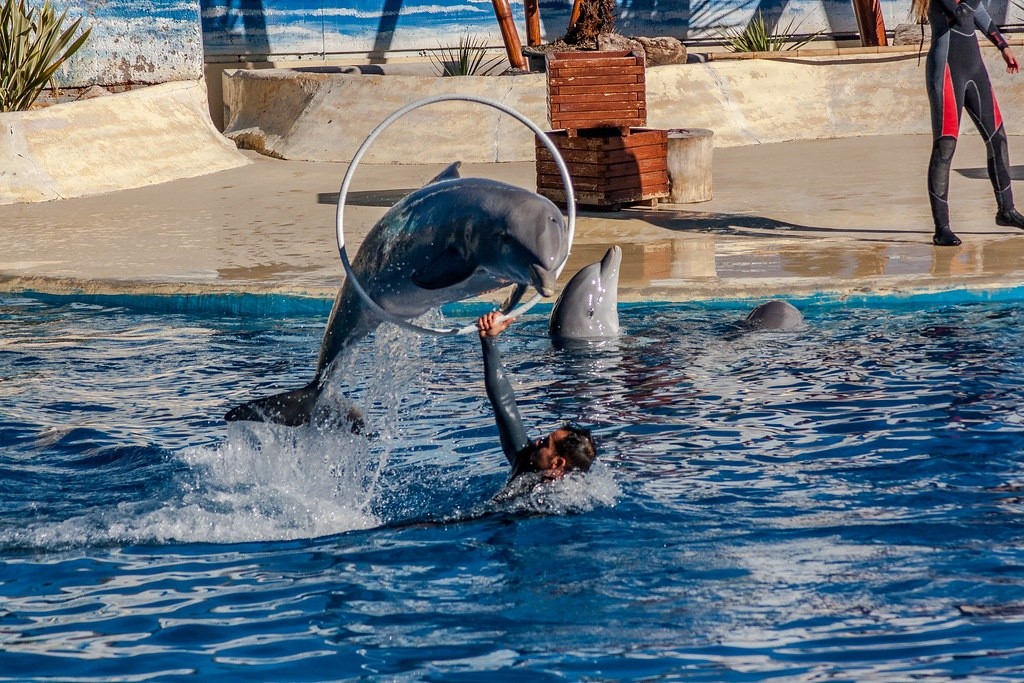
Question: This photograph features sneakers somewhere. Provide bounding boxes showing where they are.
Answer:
[933,226,962,246]
[995,203,1024,229]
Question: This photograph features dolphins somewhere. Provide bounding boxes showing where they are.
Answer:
[549,245,623,338]
[224,161,568,436]
[744,298,809,332]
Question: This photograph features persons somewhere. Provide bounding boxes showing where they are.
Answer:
[477,311,597,487]
[905,0,1024,247]
